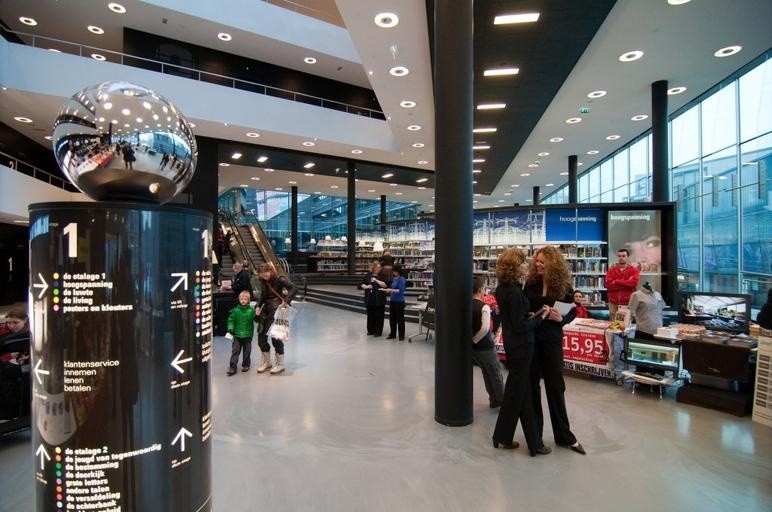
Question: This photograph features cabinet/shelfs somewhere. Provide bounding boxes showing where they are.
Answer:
[318,241,606,307]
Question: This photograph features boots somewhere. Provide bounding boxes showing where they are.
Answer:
[270,353,285,374]
[256,350,272,373]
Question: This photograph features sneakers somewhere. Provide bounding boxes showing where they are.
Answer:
[241,366,248,372]
[227,367,236,374]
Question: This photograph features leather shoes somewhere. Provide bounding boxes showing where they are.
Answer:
[399,338,404,340]
[386,336,396,339]
[570,442,586,454]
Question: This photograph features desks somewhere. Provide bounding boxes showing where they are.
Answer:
[496,317,615,382]
[679,332,758,419]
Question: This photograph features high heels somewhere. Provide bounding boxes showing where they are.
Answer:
[530,447,551,456]
[493,438,519,449]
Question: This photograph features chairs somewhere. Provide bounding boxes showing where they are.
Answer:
[408,295,435,342]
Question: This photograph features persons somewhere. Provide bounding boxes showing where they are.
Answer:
[68,140,178,170]
[628,281,666,394]
[574,290,591,318]
[215,227,298,375]
[604,248,640,322]
[492,248,552,457]
[357,249,406,341]
[522,245,587,456]
[610,211,661,273]
[0,308,30,366]
[473,276,506,408]
[67,249,210,512]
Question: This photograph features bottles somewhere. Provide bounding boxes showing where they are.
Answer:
[410,270,430,288]
[567,259,607,303]
[326,260,342,269]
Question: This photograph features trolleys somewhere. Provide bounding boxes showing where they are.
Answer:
[615,334,629,387]
